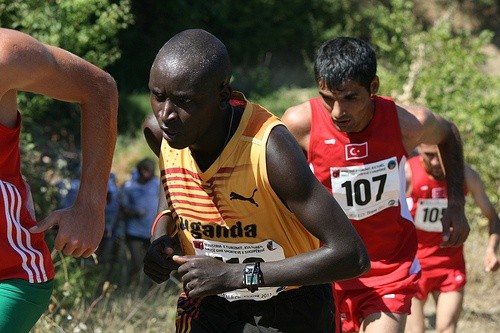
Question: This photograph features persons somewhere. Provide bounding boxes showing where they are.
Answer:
[56,157,161,277]
[143,28,370,333]
[0,27,117,333]
[281,36,470,333]
[405,143,500,333]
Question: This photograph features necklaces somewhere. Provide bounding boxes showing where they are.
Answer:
[188,104,236,189]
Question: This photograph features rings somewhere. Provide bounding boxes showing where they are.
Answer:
[186,283,191,291]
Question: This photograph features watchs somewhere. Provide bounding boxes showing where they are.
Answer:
[243,261,263,293]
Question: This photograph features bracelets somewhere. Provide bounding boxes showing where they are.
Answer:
[151,209,178,242]
